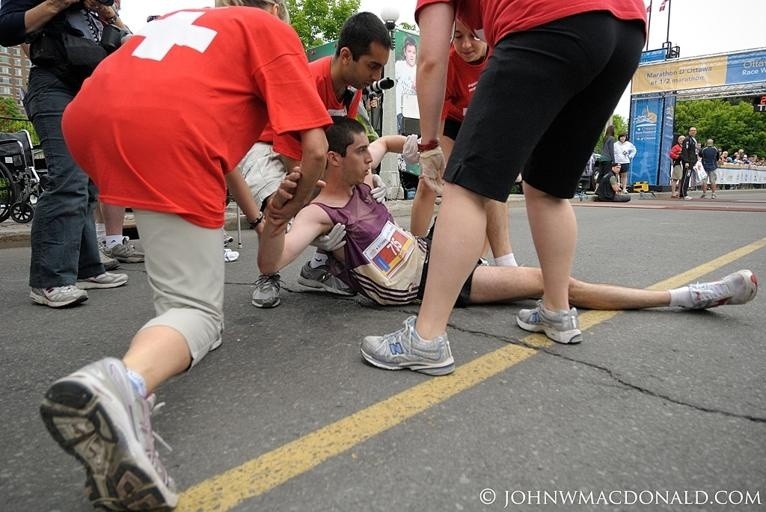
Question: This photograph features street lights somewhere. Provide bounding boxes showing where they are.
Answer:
[372,6,402,169]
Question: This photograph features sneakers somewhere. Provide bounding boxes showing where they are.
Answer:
[359,316,455,377]
[712,193,720,199]
[252,269,286,308]
[296,259,357,297]
[75,230,146,289]
[516,300,582,344]
[688,270,760,309]
[29,285,87,307]
[701,194,708,199]
[38,356,180,511]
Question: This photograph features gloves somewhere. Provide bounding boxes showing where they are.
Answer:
[369,174,388,204]
[402,134,419,165]
[310,222,348,251]
[414,138,450,196]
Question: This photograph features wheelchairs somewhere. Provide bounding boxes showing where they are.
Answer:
[0,126,46,224]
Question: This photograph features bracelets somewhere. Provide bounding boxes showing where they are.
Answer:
[415,139,443,154]
[247,208,266,232]
[102,13,119,24]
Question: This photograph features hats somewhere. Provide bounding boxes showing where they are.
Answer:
[611,163,621,166]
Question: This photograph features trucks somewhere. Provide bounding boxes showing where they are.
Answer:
[575,153,605,194]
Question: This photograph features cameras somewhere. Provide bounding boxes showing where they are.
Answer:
[90,0,116,6]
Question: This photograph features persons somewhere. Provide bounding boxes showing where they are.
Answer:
[235,9,395,312]
[254,112,759,318]
[594,119,765,202]
[39,2,336,512]
[396,37,420,139]
[78,0,147,273]
[1,1,131,309]
[398,13,522,266]
[359,0,650,378]
